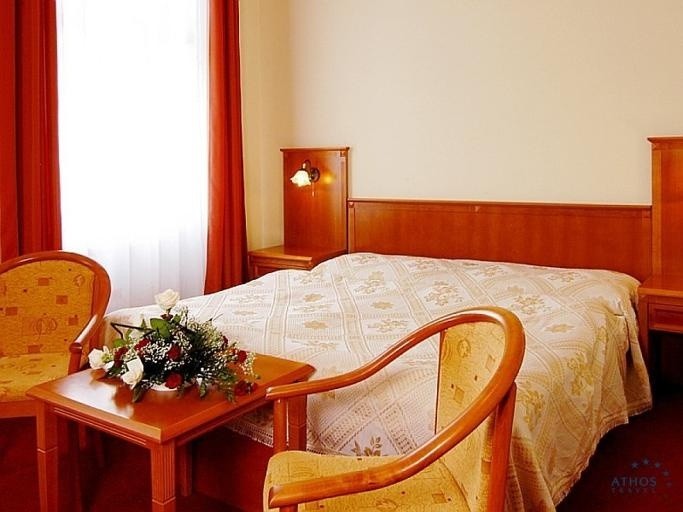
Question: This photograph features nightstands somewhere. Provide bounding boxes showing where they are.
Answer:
[249,244,346,283]
[637,274,682,420]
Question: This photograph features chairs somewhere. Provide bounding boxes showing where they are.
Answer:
[263,307,525,512]
[0,251,110,512]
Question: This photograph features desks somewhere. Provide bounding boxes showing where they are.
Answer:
[26,346,316,511]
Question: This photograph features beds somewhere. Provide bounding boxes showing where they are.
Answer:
[101,252,640,512]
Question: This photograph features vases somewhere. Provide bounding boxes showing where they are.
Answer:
[144,374,196,393]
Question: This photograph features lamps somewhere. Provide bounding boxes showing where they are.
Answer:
[289,160,321,189]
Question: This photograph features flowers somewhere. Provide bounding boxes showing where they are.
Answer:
[87,297,258,407]
